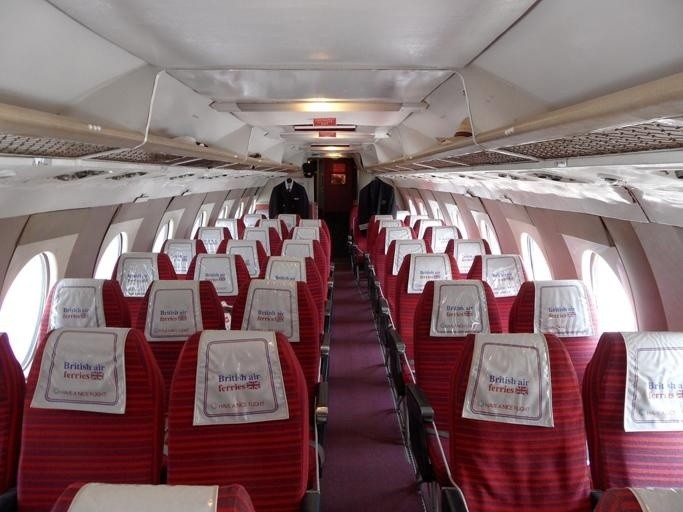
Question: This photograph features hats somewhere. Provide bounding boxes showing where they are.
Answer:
[302,163,314,178]
